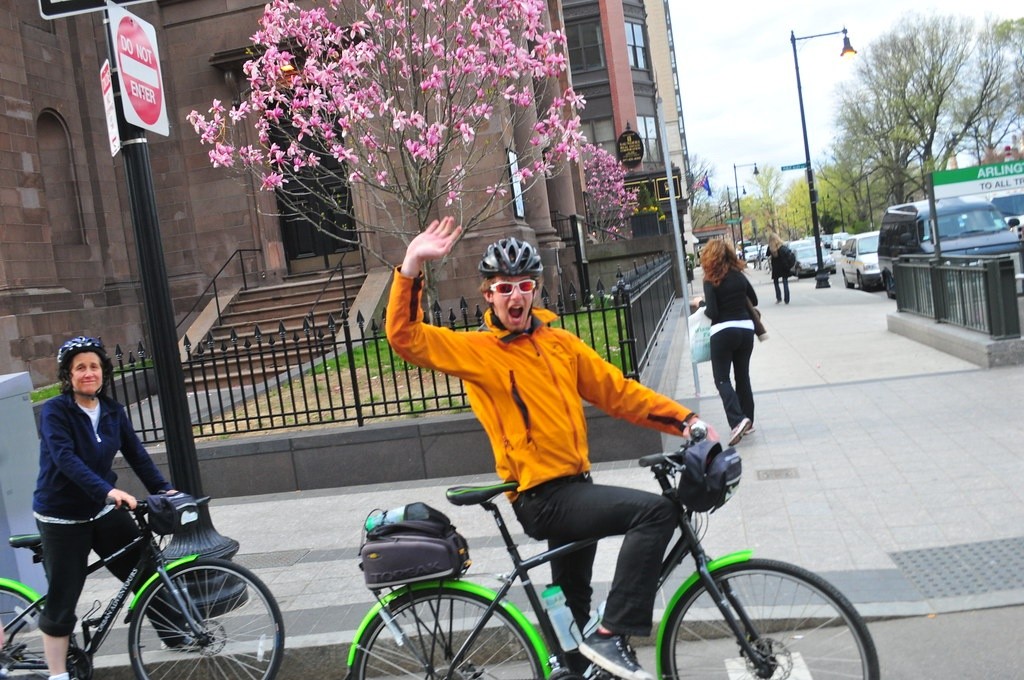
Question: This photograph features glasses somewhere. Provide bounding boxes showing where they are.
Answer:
[487,279,537,295]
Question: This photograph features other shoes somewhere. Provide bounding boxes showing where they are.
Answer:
[743,422,756,435]
[726,416,752,446]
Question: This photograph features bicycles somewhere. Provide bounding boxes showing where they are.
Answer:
[345,414,882,680]
[0,495,284,680]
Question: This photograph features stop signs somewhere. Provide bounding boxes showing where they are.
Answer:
[106,1,169,137]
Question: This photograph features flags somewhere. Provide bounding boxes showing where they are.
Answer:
[701,173,713,198]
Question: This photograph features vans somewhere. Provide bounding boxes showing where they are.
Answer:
[878,191,1024,300]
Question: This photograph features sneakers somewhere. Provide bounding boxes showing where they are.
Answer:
[159,627,206,651]
[578,631,655,680]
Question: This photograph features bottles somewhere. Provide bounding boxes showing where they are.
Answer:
[582,602,606,637]
[542,584,584,651]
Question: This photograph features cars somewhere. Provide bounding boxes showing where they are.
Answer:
[736,231,884,292]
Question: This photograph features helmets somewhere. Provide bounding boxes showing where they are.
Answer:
[477,237,544,278]
[56,335,114,376]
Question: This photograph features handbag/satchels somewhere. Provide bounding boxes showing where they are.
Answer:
[686,305,713,364]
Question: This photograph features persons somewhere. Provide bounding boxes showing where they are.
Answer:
[32,336,207,679]
[693,239,758,446]
[387,216,719,680]
[767,233,789,305]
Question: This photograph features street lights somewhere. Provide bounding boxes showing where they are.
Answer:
[726,185,747,248]
[734,163,759,266]
[790,25,859,289]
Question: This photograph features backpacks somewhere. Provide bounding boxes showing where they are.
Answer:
[777,244,795,270]
